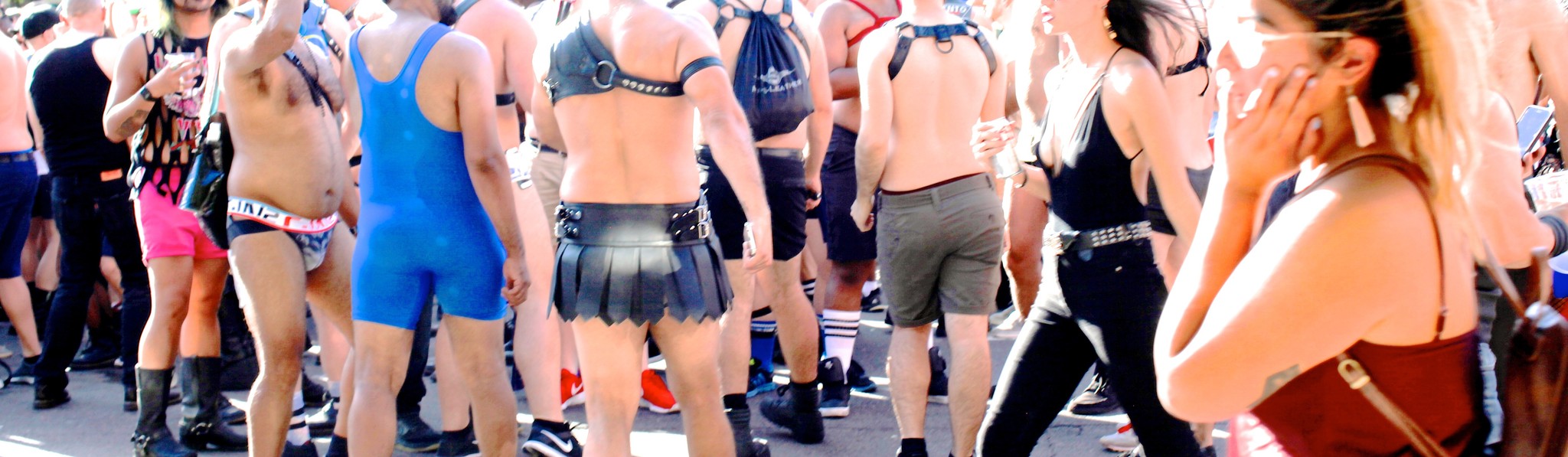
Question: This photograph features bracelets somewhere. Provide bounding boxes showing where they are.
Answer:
[1012,164,1029,188]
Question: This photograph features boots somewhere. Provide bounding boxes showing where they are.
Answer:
[178,353,249,454]
[131,363,200,456]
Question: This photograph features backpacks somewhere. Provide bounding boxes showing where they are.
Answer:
[731,0,815,140]
[1272,152,1568,457]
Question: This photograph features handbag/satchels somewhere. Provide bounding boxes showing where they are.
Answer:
[179,111,234,249]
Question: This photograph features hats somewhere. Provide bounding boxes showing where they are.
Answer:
[22,9,60,40]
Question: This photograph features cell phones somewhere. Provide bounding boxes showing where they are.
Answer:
[1515,104,1553,161]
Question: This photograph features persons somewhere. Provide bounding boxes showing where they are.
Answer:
[1,1,1568,457]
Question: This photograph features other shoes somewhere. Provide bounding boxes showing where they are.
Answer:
[926,346,949,404]
[737,437,770,457]
[646,333,664,364]
[861,291,888,313]
[849,373,877,393]
[989,305,1025,337]
[0,341,184,410]
[862,287,881,306]
[895,443,928,457]
[1119,443,1146,457]
[987,384,997,405]
[204,345,482,457]
[1201,444,1216,457]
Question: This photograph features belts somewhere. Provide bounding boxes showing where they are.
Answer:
[1057,220,1153,252]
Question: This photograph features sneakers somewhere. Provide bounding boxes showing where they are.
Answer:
[815,357,852,416]
[561,368,585,409]
[747,359,776,398]
[521,424,584,457]
[1066,372,1120,416]
[638,370,681,413]
[759,384,825,444]
[1099,422,1140,450]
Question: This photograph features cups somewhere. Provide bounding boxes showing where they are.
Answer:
[164,51,207,99]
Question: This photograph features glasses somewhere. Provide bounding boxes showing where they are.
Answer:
[1229,20,1359,66]
